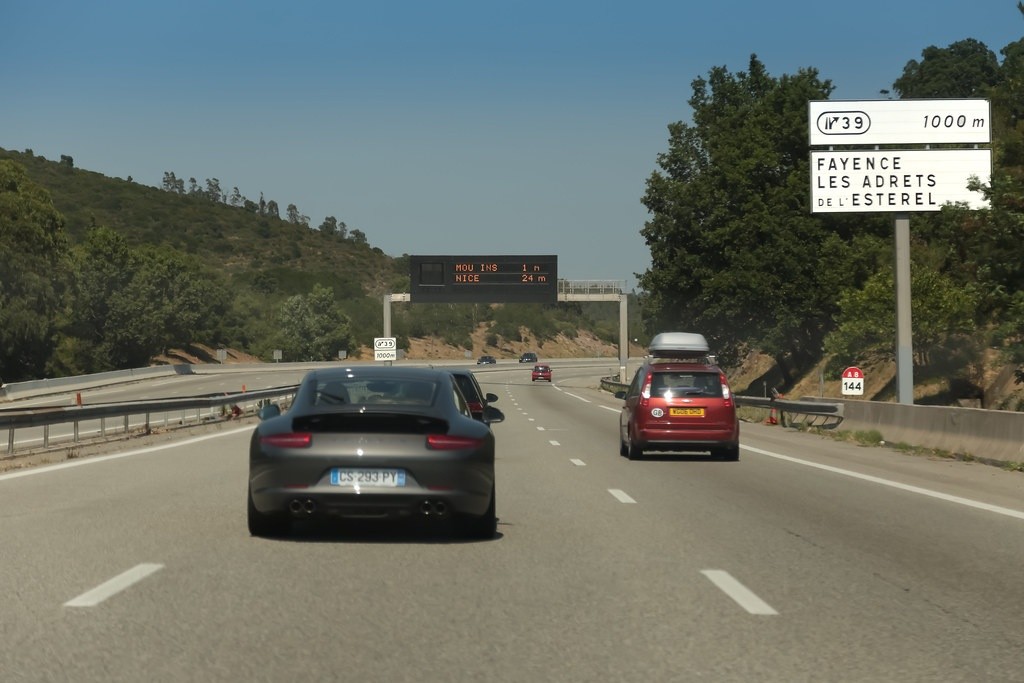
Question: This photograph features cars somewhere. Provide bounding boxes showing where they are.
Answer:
[531,364,552,382]
[519,353,538,363]
[442,367,500,429]
[476,356,497,366]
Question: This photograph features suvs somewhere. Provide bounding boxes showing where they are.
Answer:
[612,331,742,462]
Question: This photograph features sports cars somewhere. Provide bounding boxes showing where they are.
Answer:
[246,365,505,540]
